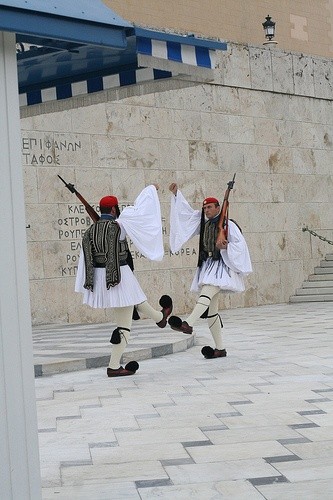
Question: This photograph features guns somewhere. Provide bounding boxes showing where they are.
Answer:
[217,172,236,242]
[58,174,100,223]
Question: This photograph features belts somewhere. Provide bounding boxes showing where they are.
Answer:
[93,259,128,267]
[206,252,213,257]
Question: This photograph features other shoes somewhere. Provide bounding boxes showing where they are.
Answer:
[107,361,139,377]
[156,295,173,328]
[201,346,226,359]
[168,316,193,334]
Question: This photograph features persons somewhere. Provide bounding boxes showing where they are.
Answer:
[75,183,173,378]
[168,183,246,359]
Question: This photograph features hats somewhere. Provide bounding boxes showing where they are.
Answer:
[203,198,219,205]
[99,196,118,206]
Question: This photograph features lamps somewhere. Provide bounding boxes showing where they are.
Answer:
[261,14,279,47]
